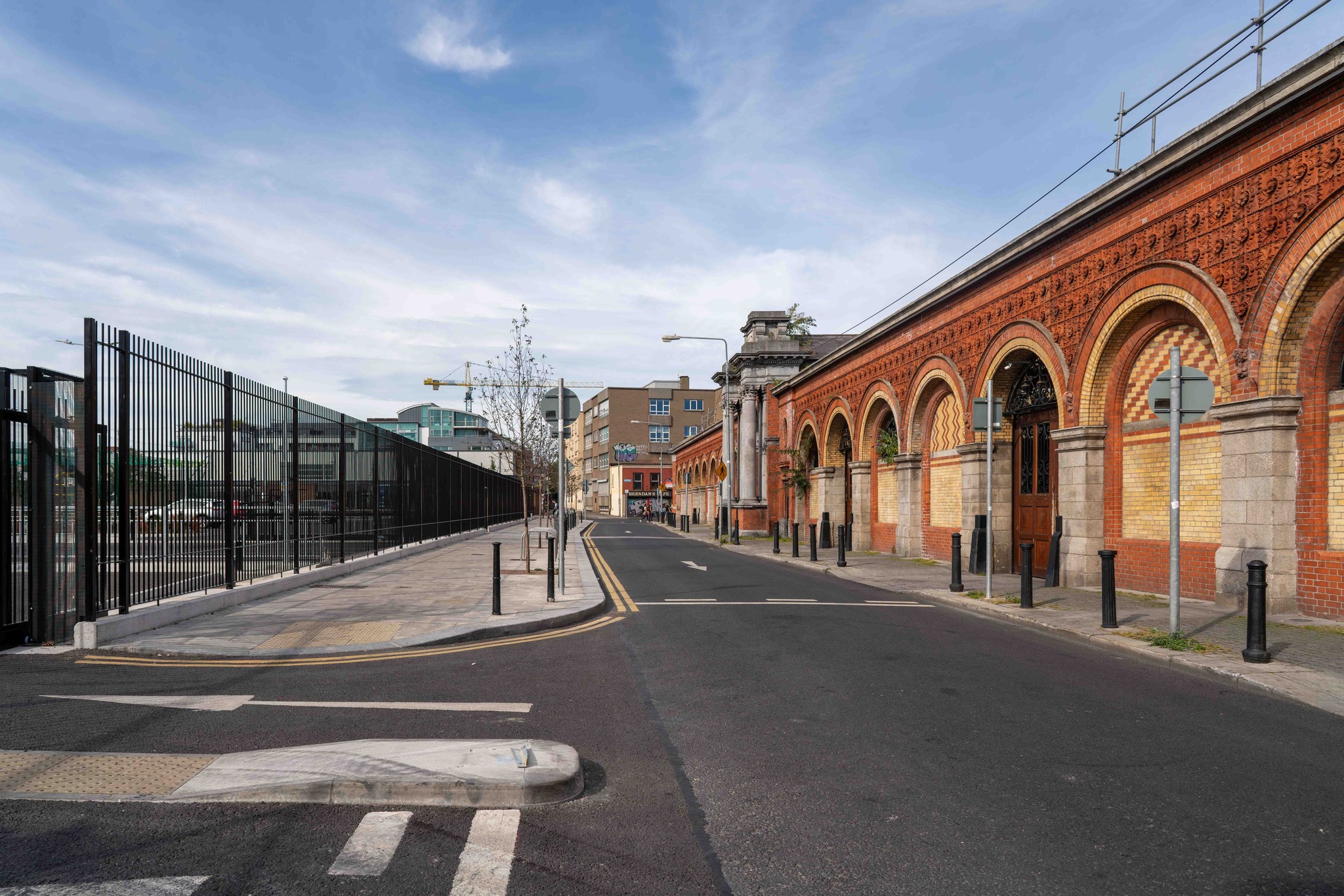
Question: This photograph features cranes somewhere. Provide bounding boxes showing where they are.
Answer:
[423,360,603,414]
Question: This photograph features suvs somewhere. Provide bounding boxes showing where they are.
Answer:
[144,500,226,531]
[280,496,339,526]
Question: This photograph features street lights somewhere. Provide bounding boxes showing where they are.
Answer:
[662,334,732,543]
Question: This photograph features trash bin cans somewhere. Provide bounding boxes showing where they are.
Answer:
[554,510,568,542]
[568,508,576,527]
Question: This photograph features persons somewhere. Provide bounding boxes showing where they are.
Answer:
[664,503,671,521]
[649,501,653,522]
[639,504,650,523]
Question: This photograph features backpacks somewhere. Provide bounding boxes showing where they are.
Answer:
[647,505,651,511]
[555,503,559,510]
[642,506,646,513]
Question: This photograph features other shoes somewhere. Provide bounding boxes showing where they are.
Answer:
[650,519,653,521]
[649,520,650,522]
[646,521,650,523]
[639,520,642,522]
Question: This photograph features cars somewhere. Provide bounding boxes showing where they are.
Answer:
[233,500,248,523]
